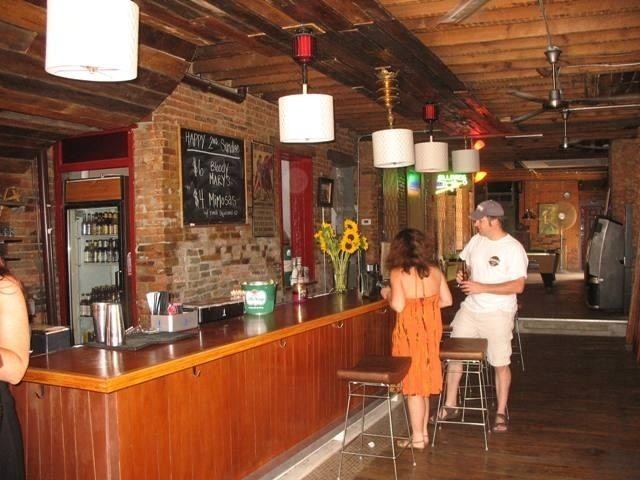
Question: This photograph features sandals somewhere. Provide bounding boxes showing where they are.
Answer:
[397,436,429,450]
[493,414,508,433]
[428,408,460,424]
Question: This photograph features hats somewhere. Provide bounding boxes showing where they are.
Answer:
[468,200,504,220]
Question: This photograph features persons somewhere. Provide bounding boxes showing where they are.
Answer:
[0,257,32,479]
[381,228,453,450]
[429,200,530,434]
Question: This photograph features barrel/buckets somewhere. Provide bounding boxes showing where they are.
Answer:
[241,279,279,316]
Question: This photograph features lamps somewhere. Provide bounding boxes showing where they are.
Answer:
[278,29,480,174]
[44,0,140,83]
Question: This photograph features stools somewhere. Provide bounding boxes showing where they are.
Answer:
[335,356,416,479]
[432,338,491,450]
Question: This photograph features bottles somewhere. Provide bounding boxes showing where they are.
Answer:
[77,211,120,237]
[78,285,120,316]
[292,259,304,285]
[291,276,307,302]
[83,240,120,263]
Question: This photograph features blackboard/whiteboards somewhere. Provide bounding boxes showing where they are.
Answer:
[178,125,249,228]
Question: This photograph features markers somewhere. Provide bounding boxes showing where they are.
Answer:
[190,223,196,227]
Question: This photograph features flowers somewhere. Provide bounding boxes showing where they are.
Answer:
[313,220,368,290]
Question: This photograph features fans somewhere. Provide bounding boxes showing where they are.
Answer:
[504,47,604,123]
[550,202,577,274]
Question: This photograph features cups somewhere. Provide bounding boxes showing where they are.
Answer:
[89,302,126,347]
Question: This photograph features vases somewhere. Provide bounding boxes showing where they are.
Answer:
[330,260,350,296]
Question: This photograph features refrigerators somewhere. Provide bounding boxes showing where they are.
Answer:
[54,128,134,349]
